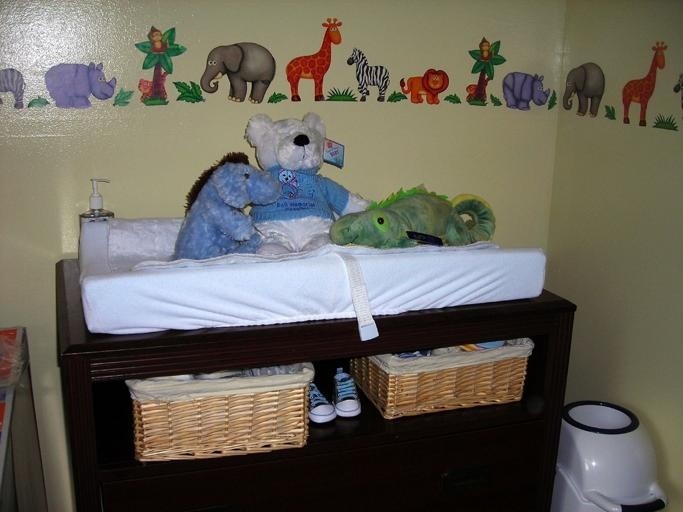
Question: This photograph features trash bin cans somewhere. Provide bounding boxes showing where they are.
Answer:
[550,400,668,512]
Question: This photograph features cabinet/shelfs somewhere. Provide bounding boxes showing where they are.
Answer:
[55,256,576,512]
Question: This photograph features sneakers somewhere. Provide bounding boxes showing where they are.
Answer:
[307,371,362,424]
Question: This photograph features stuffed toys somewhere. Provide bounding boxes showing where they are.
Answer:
[172,149,282,258]
[329,183,497,247]
[244,112,372,257]
